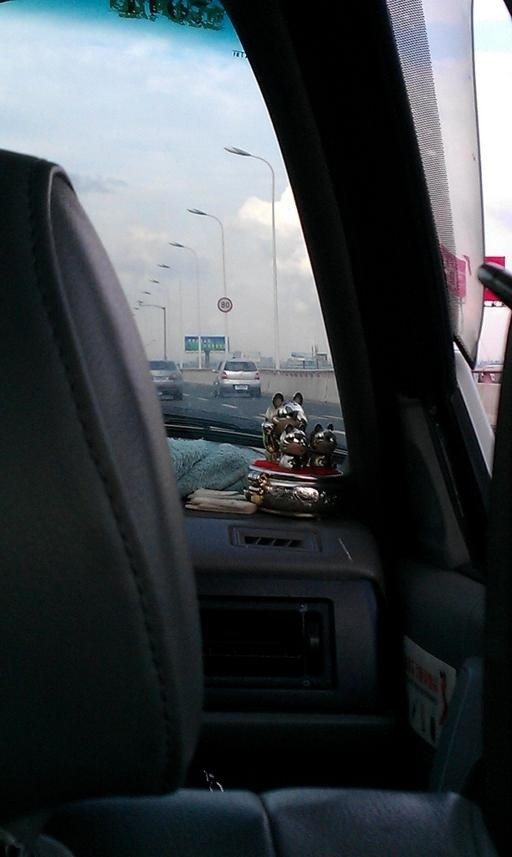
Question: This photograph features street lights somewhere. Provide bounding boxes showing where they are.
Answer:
[138,240,201,369]
[187,207,228,354]
[223,144,280,370]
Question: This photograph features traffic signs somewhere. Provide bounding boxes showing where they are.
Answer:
[218,297,232,312]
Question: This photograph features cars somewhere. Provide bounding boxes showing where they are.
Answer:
[212,360,261,397]
[478,365,503,383]
[149,362,184,400]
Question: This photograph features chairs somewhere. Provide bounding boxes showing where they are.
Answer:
[0,150,501,856]
